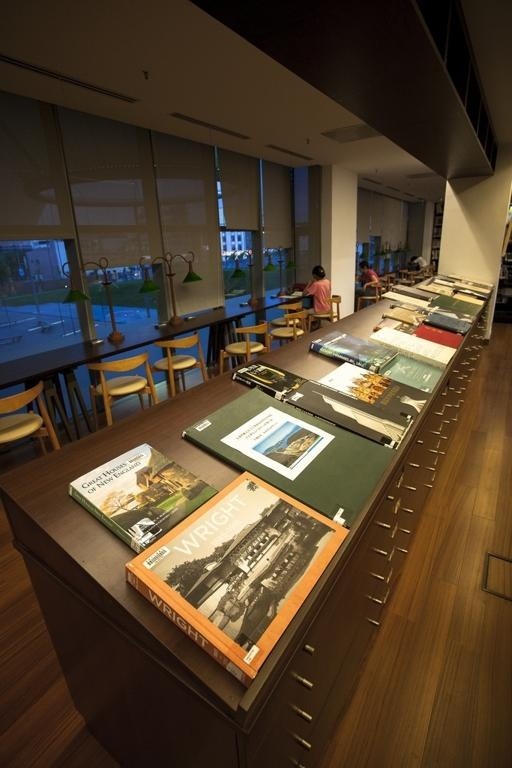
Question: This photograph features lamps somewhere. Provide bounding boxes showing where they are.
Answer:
[58,244,297,346]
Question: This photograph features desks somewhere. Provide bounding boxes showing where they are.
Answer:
[0,275,497,764]
[0,282,299,440]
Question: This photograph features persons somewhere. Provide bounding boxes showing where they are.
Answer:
[408,255,428,276]
[300,264,331,324]
[354,259,381,297]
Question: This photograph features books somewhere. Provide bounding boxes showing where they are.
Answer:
[66,441,218,555]
[123,469,352,689]
[283,272,494,449]
[230,359,309,403]
[181,386,396,530]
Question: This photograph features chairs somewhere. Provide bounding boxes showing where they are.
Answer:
[357,263,420,311]
[215,293,343,376]
[87,330,210,429]
[1,382,60,457]
